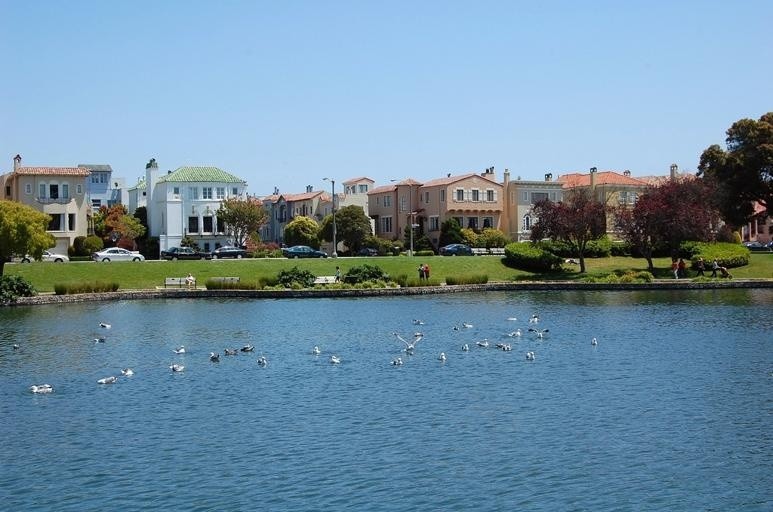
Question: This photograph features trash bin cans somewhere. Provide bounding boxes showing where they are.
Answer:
[393,247,400,256]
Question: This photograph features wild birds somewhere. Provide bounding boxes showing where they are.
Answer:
[497,315,550,361]
[28,384,53,394]
[169,362,185,372]
[454,322,488,351]
[12,344,20,351]
[392,318,426,367]
[591,337,598,345]
[313,344,321,357]
[93,338,105,344]
[257,356,268,367]
[437,352,447,365]
[99,321,112,329]
[170,345,186,355]
[328,356,341,365]
[95,366,134,385]
[209,343,255,363]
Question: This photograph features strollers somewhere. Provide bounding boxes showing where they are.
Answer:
[719,266,733,280]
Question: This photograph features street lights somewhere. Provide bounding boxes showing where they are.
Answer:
[390,179,413,257]
[322,177,337,258]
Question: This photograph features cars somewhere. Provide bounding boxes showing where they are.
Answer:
[415,246,434,256]
[283,246,327,259]
[744,241,773,251]
[161,246,247,261]
[92,247,145,262]
[13,250,69,263]
[439,243,475,256]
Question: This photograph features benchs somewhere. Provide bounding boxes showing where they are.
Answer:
[164,277,197,289]
[211,276,240,283]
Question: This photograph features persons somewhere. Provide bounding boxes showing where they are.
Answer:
[672,258,679,279]
[711,258,721,278]
[185,272,194,290]
[334,266,340,284]
[422,264,430,281]
[415,263,424,280]
[679,259,687,278]
[696,257,705,276]
